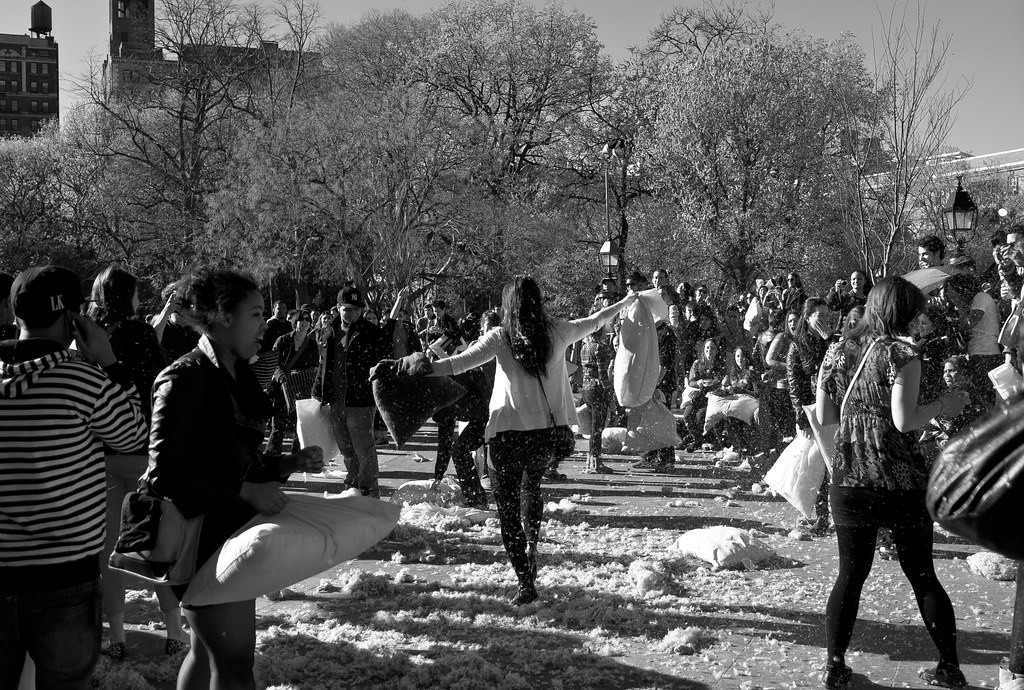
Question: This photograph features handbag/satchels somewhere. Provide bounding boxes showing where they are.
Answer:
[998,295,1024,348]
[108,485,205,586]
[927,400,1024,562]
[988,362,1024,406]
[555,425,575,461]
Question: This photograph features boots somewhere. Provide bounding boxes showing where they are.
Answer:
[811,492,831,533]
[586,453,614,474]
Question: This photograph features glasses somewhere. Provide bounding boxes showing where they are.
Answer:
[298,317,310,322]
[625,282,636,287]
[1005,238,1021,247]
[697,289,707,294]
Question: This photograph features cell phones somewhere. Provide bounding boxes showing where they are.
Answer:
[72,318,88,344]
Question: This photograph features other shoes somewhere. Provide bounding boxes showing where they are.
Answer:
[918,662,967,689]
[821,665,852,689]
[166,639,190,656]
[542,470,567,484]
[994,666,1024,690]
[101,642,128,662]
[879,544,897,559]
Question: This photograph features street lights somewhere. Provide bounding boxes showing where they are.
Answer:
[942,175,978,268]
[599,234,620,279]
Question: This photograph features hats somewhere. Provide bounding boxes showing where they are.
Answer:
[338,287,367,308]
[11,265,100,319]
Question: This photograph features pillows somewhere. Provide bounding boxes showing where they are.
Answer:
[179,488,403,606]
[576,403,610,435]
[703,391,760,437]
[743,297,762,336]
[666,526,777,568]
[599,427,628,453]
[295,399,341,465]
[763,423,841,519]
[388,478,461,508]
[614,288,668,408]
[368,352,468,450]
[802,402,840,475]
[621,390,682,452]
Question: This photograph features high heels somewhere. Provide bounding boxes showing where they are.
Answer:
[527,542,537,582]
[511,573,539,606]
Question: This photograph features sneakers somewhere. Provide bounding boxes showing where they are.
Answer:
[655,463,675,473]
[632,460,656,468]
[375,437,389,447]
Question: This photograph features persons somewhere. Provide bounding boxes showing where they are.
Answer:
[256,285,410,460]
[817,274,972,690]
[321,288,383,498]
[89,269,194,660]
[580,267,823,537]
[152,269,324,690]
[0,267,147,690]
[152,274,495,507]
[427,278,638,607]
[786,225,1024,537]
